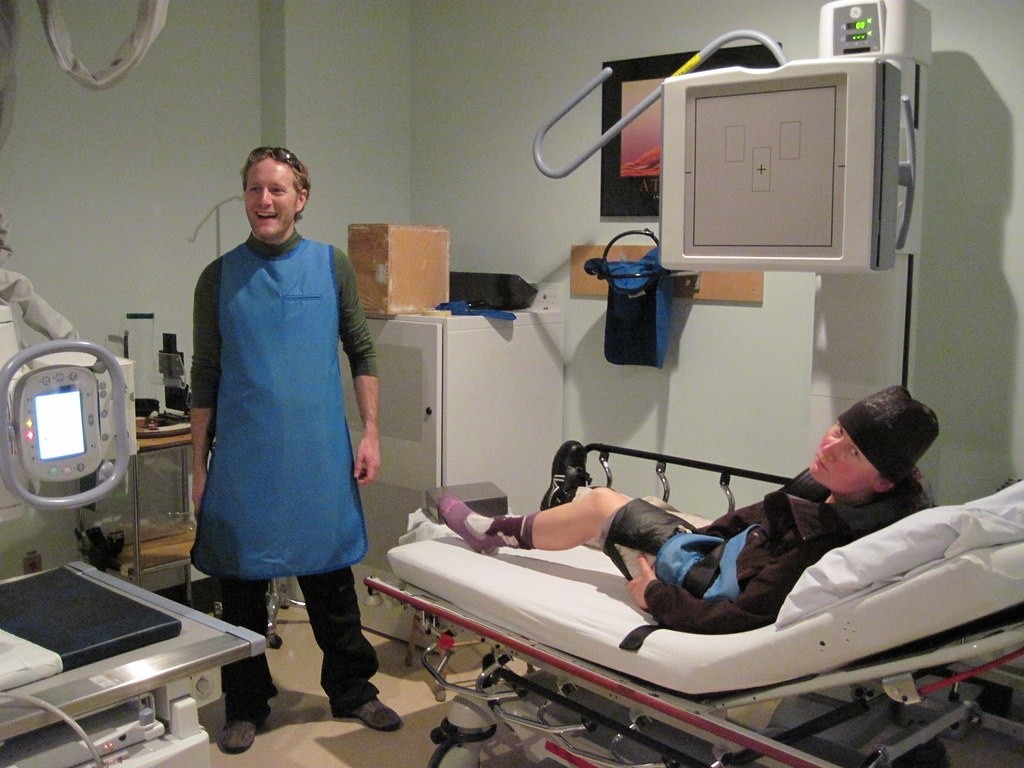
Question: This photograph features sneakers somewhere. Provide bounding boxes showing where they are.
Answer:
[541,441,591,511]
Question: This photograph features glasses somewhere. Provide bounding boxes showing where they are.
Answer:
[248,148,300,171]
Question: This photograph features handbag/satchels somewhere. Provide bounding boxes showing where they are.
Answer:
[584,247,671,369]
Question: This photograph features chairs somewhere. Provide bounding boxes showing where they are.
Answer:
[361,473,1024,768]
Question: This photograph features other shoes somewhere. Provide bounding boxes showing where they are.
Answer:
[217,721,254,755]
[352,696,405,731]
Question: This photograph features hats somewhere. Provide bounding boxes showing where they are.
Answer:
[839,385,938,488]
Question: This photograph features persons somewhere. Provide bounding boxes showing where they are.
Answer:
[437,384,940,632]
[189,148,402,755]
